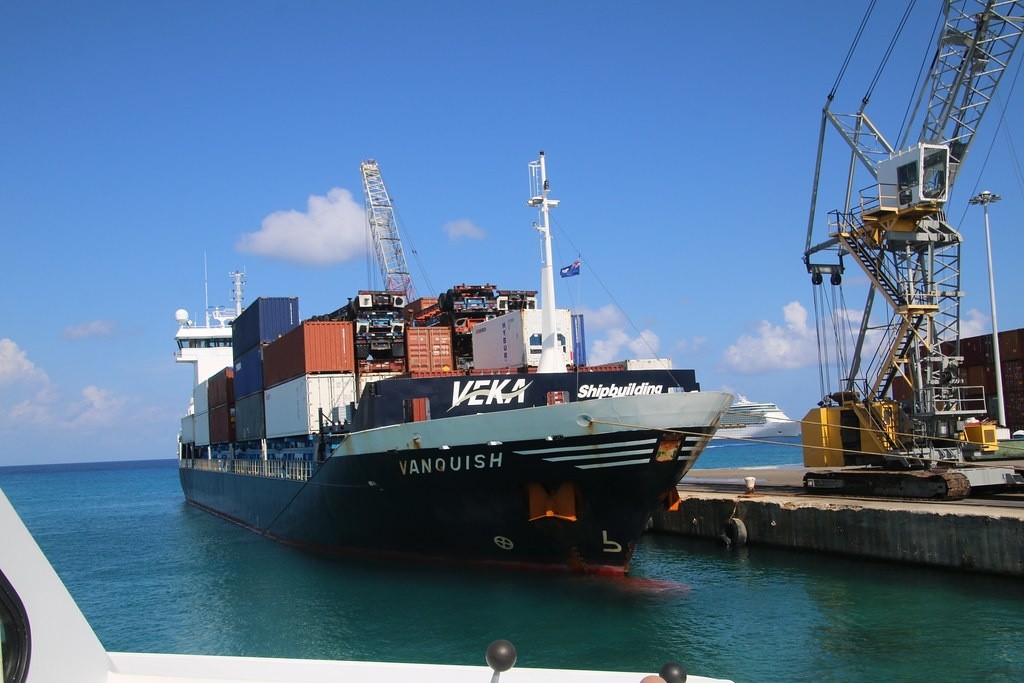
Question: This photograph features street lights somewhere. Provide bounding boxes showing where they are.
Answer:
[968,191,1008,428]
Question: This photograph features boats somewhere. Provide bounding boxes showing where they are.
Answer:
[173,152,735,583]
[0,488,733,683]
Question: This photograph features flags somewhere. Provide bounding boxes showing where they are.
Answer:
[560,260,580,278]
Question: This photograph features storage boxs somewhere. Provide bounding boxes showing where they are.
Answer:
[891,327,1024,439]
[179,296,670,448]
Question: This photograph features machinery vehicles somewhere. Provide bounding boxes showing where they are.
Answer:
[801,1,1024,501]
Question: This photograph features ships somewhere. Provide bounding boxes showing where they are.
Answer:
[712,392,801,440]
